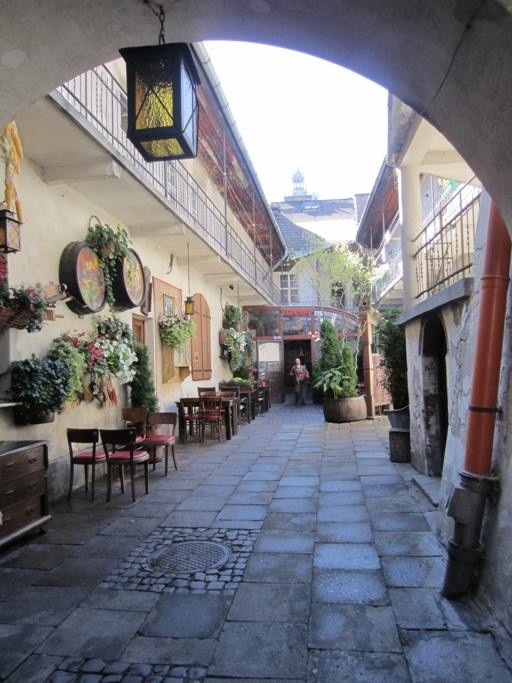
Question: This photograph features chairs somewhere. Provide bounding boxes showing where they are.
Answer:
[65,379,270,503]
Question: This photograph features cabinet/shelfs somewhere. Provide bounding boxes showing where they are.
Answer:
[1,439,53,547]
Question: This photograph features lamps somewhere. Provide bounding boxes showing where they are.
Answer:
[116,0,207,163]
[183,242,198,314]
[0,209,23,253]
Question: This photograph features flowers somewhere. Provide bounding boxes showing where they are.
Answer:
[0,280,75,333]
[46,318,137,409]
[157,312,197,352]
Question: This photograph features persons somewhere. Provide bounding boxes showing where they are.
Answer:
[288,356,311,404]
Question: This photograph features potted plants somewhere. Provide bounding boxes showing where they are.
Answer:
[220,303,255,373]
[82,214,135,328]
[312,366,370,422]
[2,353,68,427]
